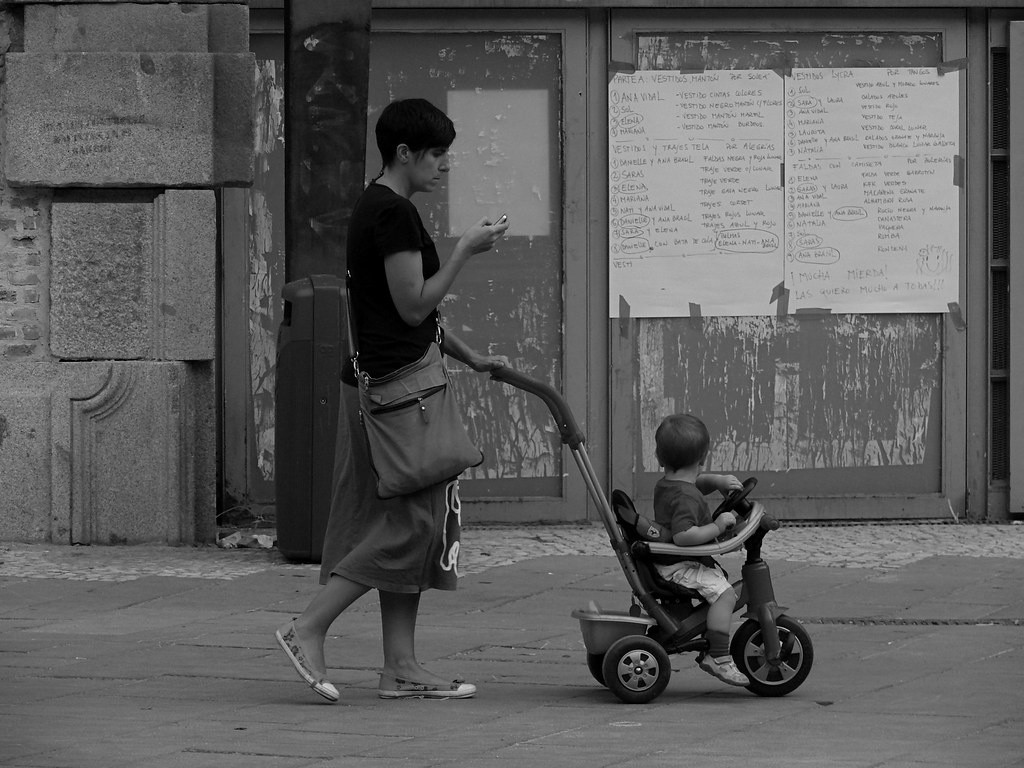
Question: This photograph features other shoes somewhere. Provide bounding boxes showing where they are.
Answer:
[275,620,341,702]
[699,654,750,687]
[377,671,477,698]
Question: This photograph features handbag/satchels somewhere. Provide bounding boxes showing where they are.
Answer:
[352,342,485,498]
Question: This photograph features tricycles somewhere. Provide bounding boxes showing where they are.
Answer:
[491,363,814,704]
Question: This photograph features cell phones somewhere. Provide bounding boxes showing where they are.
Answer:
[494,215,507,226]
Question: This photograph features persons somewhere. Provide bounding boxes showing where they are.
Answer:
[654,412,749,686]
[275,98,510,700]
